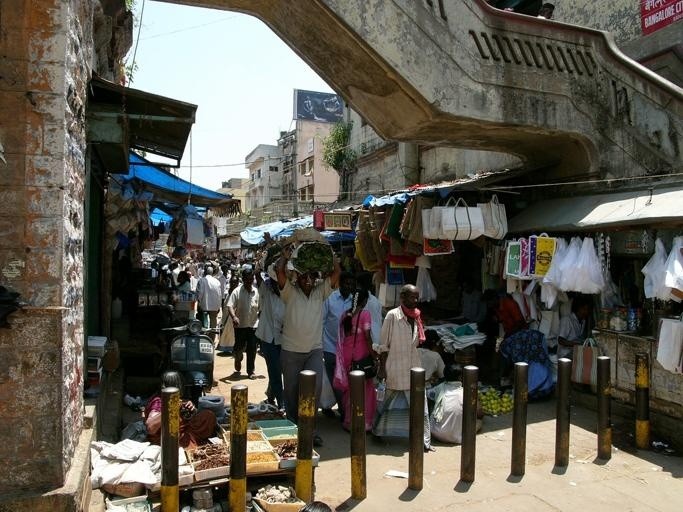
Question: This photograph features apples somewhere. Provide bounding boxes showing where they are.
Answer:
[478,388,514,415]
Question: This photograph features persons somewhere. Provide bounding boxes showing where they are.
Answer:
[553,294,590,388]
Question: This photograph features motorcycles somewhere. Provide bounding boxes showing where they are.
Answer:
[157,318,223,406]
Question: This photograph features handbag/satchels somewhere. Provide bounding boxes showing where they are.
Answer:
[379,283,401,307]
[571,338,597,384]
[354,194,508,271]
[503,233,556,280]
[352,357,376,378]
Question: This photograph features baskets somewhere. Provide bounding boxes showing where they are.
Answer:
[101,418,321,512]
